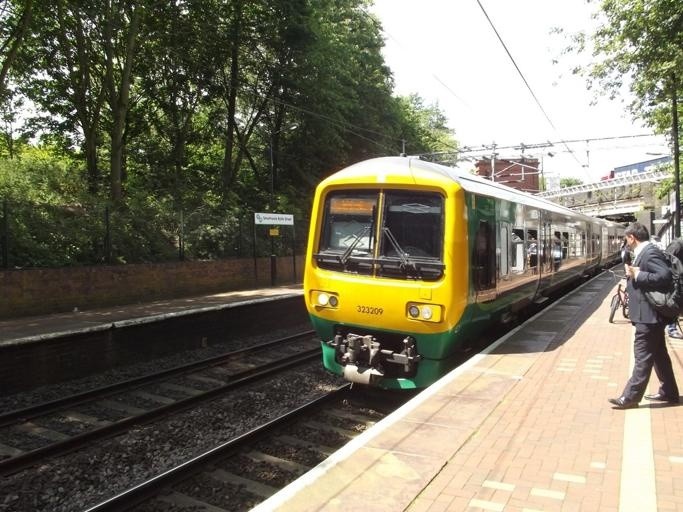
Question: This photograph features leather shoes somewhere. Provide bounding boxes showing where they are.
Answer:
[669,332,683,338]
[608,396,639,408]
[644,393,679,401]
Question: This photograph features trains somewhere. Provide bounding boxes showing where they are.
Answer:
[302,140,632,393]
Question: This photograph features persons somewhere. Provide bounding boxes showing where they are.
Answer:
[608,223,683,409]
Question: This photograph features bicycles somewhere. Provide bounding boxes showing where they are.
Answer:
[600,268,631,324]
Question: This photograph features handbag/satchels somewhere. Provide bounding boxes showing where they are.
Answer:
[640,248,683,319]
